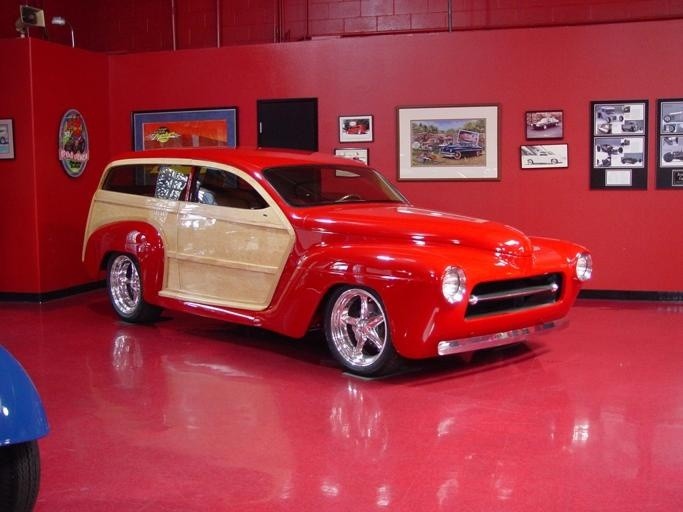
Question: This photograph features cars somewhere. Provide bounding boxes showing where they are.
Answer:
[79,148,598,376]
[523,150,563,164]
[438,142,482,160]
[596,105,683,167]
[0,343,44,512]
[529,117,559,130]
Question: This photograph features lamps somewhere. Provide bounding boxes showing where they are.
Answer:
[52,16,77,49]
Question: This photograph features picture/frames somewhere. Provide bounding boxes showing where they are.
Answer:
[654,96,682,191]
[518,143,570,171]
[131,106,244,190]
[57,108,91,178]
[587,96,649,193]
[333,146,371,179]
[1,116,18,163]
[394,101,503,184]
[521,106,567,142]
[338,113,375,144]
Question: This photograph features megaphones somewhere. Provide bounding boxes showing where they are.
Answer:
[15,5,46,33]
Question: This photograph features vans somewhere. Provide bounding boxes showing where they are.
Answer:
[346,124,366,135]
